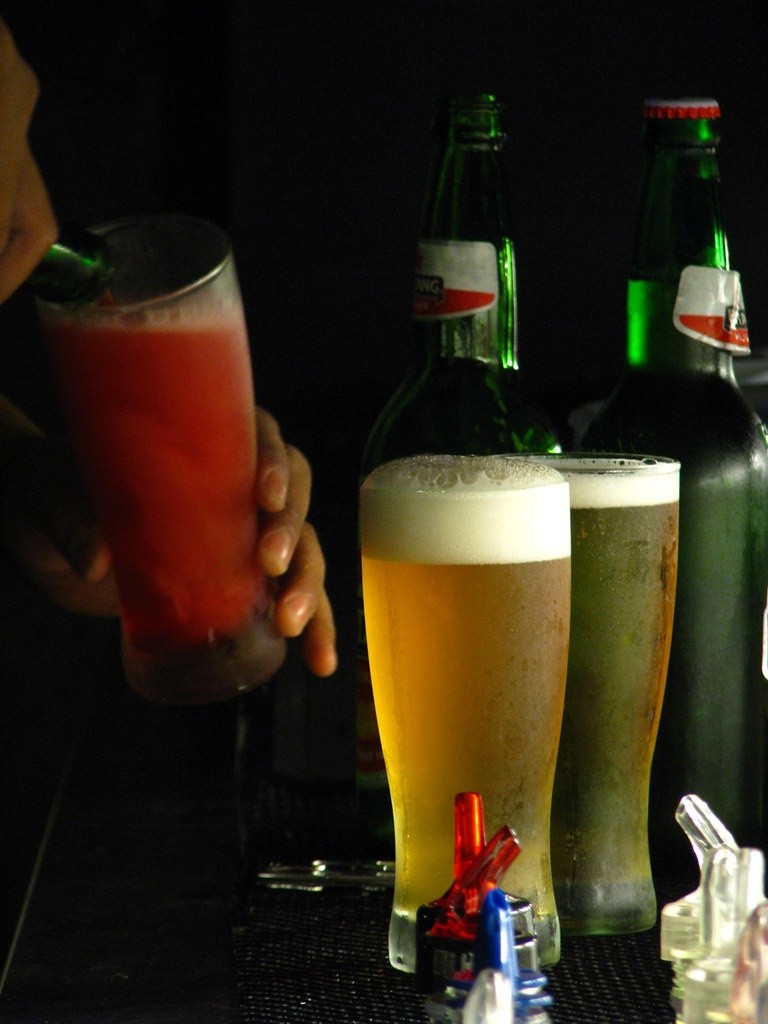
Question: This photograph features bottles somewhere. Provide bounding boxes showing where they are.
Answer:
[356,91,560,864]
[29,224,117,308]
[578,97,768,850]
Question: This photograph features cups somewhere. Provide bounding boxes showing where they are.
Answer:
[515,451,684,944]
[358,475,562,975]
[45,218,291,708]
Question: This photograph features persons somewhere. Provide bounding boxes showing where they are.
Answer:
[0,22,339,680]
